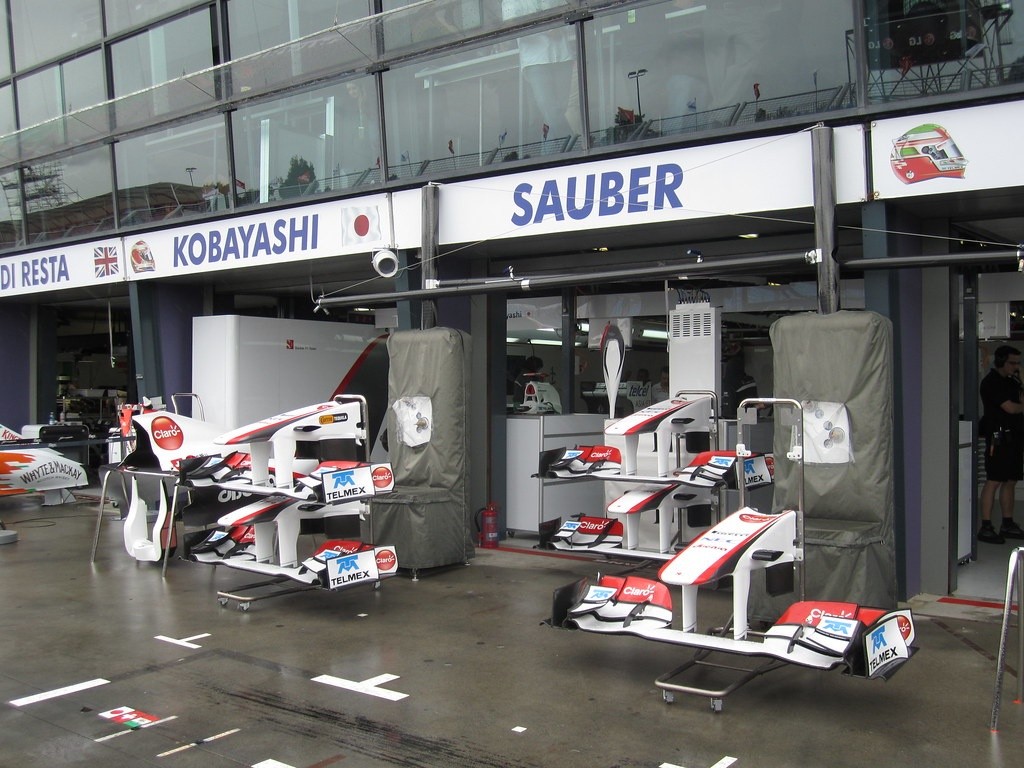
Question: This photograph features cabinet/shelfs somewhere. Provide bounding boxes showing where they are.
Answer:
[532,389,919,713]
[23,422,89,470]
[507,413,608,536]
[86,392,399,611]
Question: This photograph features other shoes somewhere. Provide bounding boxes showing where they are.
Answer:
[977,526,1005,544]
[999,522,1024,540]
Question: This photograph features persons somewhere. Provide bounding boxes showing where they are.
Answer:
[513,357,542,413]
[732,370,758,405]
[651,367,669,404]
[638,370,652,387]
[980,345,1024,544]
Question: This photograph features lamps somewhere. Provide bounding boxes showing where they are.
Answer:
[371,247,399,277]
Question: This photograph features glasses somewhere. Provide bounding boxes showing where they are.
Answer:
[1006,360,1021,365]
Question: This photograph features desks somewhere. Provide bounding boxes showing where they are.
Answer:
[150,95,337,199]
[413,4,706,168]
